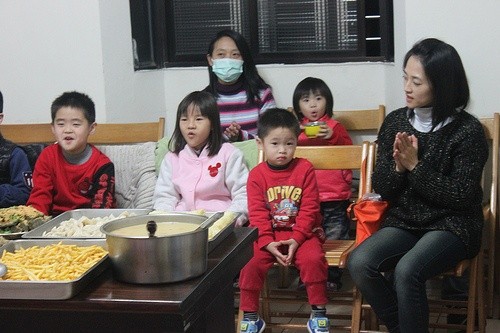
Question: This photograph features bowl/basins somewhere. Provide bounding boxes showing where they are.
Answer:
[305,122,326,137]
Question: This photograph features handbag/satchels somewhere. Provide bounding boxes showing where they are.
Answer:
[348,199,387,246]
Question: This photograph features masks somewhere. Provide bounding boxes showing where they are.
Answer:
[210,58,244,83]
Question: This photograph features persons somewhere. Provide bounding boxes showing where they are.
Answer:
[345,39,490,333]
[200,28,278,143]
[26,92,116,215]
[292,76,354,293]
[153,91,251,228]
[0,90,33,208]
[237,107,331,333]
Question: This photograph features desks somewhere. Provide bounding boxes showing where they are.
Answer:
[0,225,258,333]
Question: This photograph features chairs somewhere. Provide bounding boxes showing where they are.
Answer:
[235,105,500,333]
[155,136,263,178]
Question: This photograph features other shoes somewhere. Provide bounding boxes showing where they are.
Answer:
[240,314,266,333]
[307,314,331,333]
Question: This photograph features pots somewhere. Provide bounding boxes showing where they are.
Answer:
[101,213,208,283]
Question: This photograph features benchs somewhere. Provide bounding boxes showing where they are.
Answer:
[0,117,164,214]
